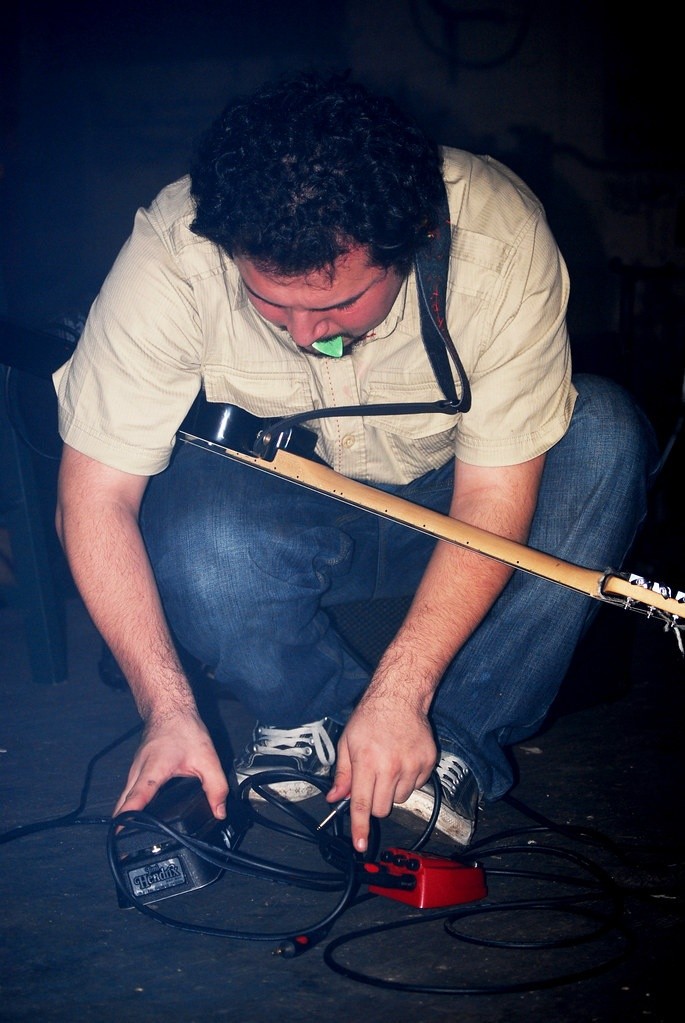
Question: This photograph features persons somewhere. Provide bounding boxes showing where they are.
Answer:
[52,77,649,852]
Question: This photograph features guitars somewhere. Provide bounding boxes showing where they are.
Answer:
[0,310,685,657]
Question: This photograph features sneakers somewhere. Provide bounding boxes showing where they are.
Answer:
[386,754,480,849]
[227,718,345,802]
